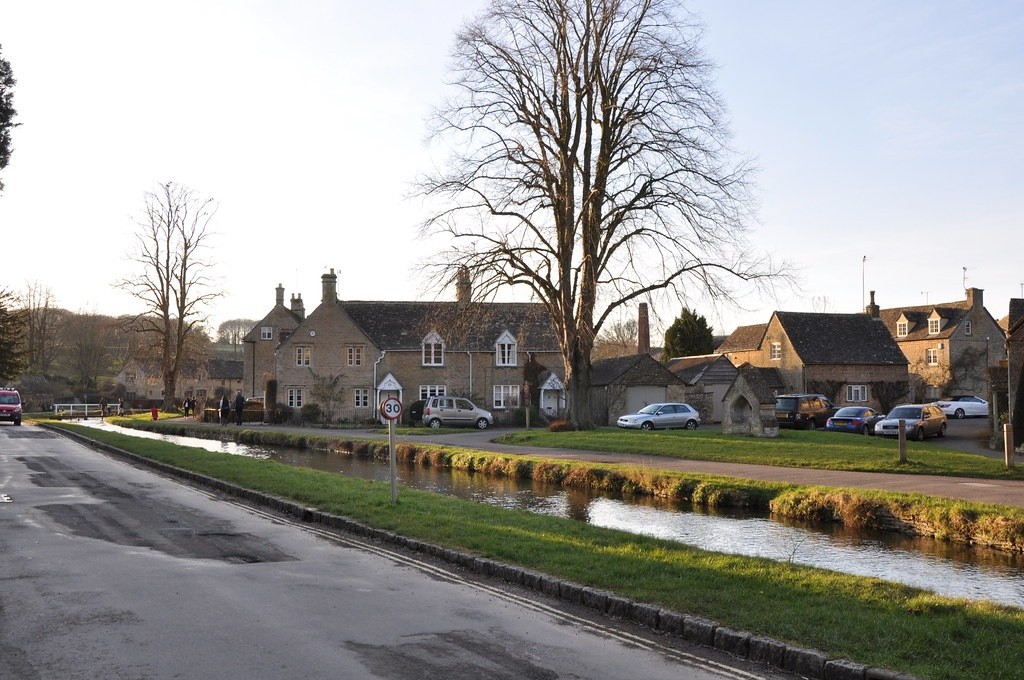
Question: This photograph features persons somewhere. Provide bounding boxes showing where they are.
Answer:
[99,397,112,416]
[219,394,230,426]
[183,396,197,419]
[231,391,247,426]
[118,398,125,417]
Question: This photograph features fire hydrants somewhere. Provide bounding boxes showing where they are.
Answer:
[151,406,160,421]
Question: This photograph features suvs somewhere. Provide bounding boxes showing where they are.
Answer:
[774,393,843,430]
[0,387,26,426]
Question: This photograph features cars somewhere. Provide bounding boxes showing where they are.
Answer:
[932,394,988,419]
[874,403,947,442]
[422,395,495,429]
[617,402,701,431]
[825,406,887,436]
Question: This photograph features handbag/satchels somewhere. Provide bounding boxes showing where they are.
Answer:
[242,401,247,407]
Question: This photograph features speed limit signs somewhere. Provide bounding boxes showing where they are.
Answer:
[382,397,402,420]
